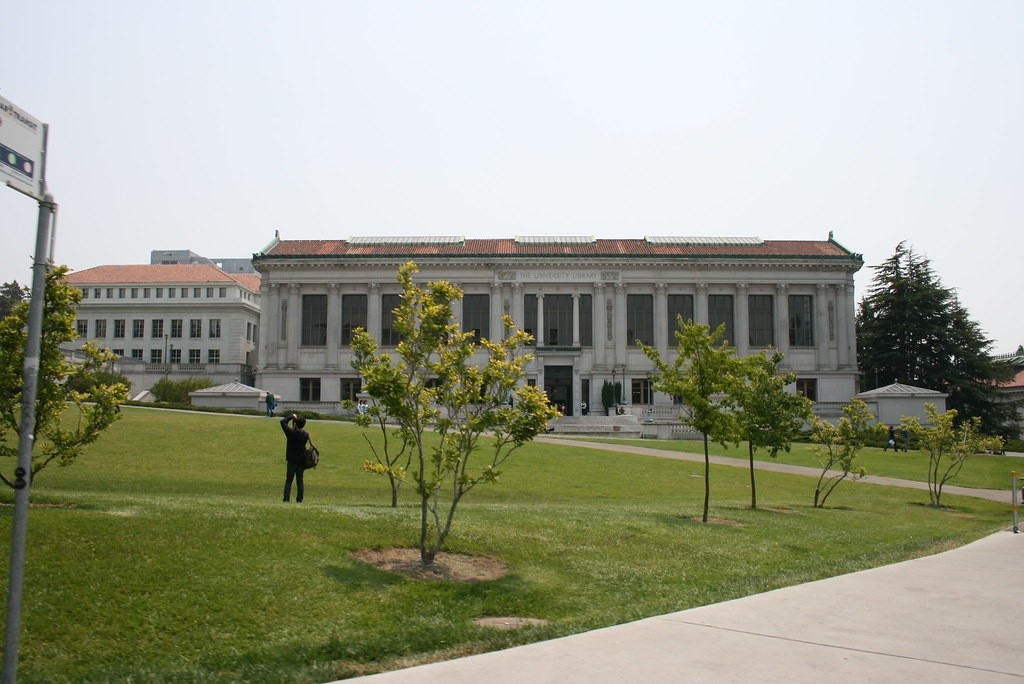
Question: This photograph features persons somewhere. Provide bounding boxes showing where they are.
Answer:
[266,391,276,417]
[883,426,898,452]
[560,403,567,414]
[358,400,369,416]
[280,413,310,503]
[999,431,1008,455]
[901,427,909,452]
[581,401,587,416]
[618,407,626,415]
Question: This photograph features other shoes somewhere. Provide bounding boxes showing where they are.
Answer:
[905,451,907,452]
[895,449,897,452]
[283,499,289,502]
[883,449,886,451]
[901,448,903,451]
[297,500,302,503]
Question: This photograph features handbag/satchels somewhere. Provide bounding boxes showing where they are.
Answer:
[303,439,319,470]
[272,404,277,406]
